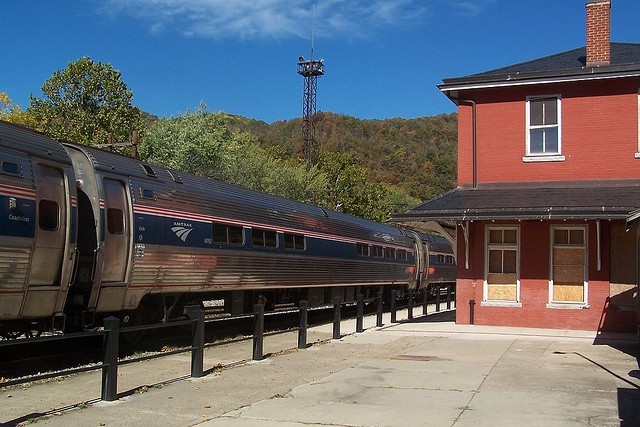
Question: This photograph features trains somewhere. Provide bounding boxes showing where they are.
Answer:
[0,114,455,348]
